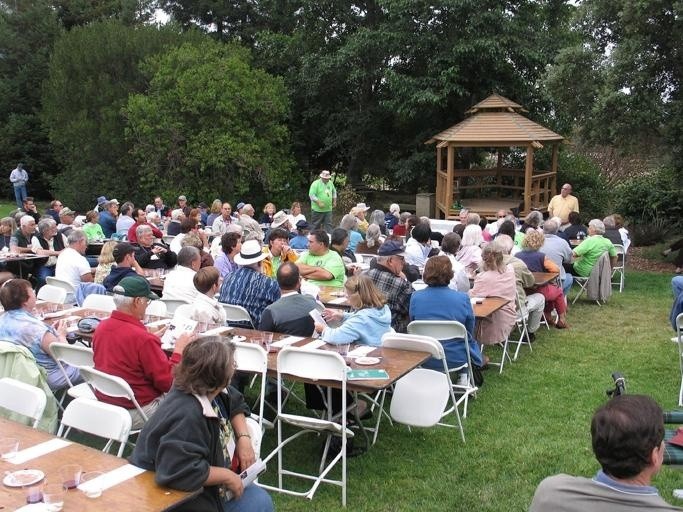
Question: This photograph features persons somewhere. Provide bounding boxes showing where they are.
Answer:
[9,162,29,208]
[308,170,337,234]
[547,183,580,232]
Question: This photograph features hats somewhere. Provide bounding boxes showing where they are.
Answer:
[377,239,406,257]
[270,210,291,229]
[58,207,75,218]
[353,202,370,212]
[319,170,332,179]
[112,241,141,258]
[177,195,185,201]
[232,239,270,266]
[296,219,310,228]
[96,196,107,206]
[111,275,159,300]
[109,198,119,205]
[236,203,244,209]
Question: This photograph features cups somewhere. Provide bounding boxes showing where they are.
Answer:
[23,465,105,511]
[248,331,291,354]
[334,343,348,355]
[0,437,18,459]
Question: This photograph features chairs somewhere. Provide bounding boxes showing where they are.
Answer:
[670,312,683,408]
[0,240,625,511]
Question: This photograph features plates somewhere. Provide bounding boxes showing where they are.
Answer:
[3,470,44,488]
[355,357,378,365]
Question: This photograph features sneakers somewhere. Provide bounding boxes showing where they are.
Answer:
[672,270,682,275]
[659,250,669,257]
[521,332,536,344]
[539,314,554,325]
[555,318,570,329]
[671,487,683,500]
[670,335,683,343]
[458,381,476,397]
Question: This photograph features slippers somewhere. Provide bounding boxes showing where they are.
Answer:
[334,406,374,427]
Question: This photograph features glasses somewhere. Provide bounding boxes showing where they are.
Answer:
[499,216,506,218]
